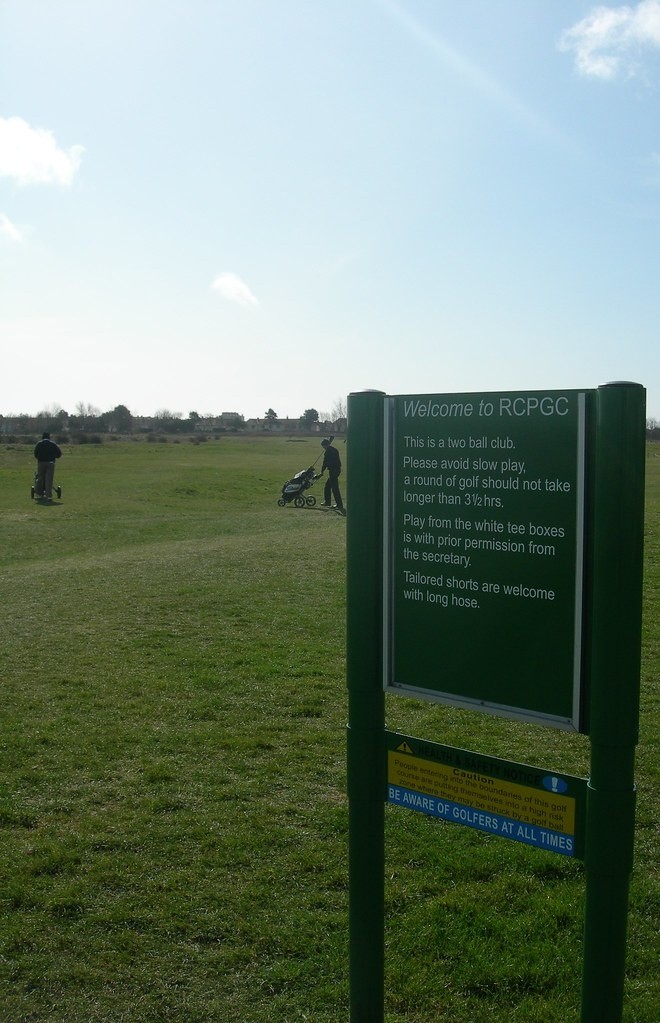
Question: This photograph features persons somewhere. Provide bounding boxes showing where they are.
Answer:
[320,439,343,510]
[34,432,62,502]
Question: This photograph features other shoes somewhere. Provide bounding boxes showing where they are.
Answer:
[321,502,331,506]
[333,504,343,508]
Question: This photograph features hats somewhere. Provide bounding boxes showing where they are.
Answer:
[321,439,330,445]
[42,432,51,439]
